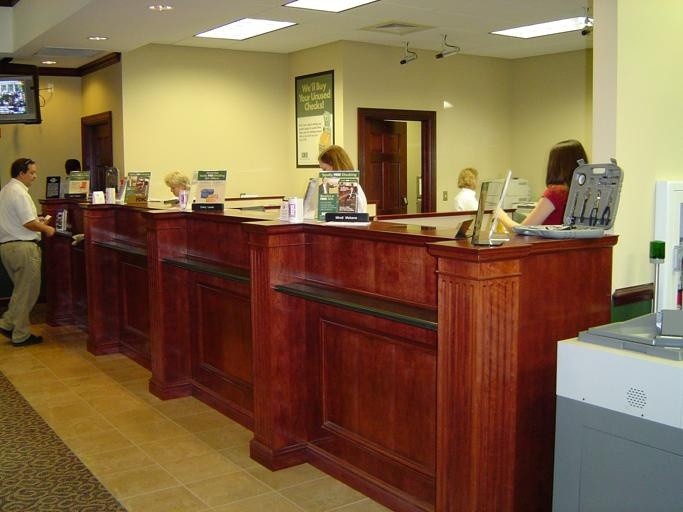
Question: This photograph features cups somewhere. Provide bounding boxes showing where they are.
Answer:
[106,187,116,205]
[179,189,189,209]
[288,197,305,224]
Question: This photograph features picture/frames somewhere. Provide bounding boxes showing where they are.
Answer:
[295,69,335,169]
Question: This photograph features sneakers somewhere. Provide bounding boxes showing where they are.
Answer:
[0,327,14,339]
[11,334,43,346]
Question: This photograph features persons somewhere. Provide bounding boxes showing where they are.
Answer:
[164,169,192,197]
[491,139,589,237]
[318,144,368,213]
[0,158,55,347]
[454,167,480,212]
[60,159,81,198]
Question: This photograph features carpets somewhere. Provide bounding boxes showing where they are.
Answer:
[0,369,128,511]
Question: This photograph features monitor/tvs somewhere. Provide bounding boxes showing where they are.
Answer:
[0,64,41,124]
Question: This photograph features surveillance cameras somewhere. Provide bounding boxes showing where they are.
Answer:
[400,53,418,65]
[435,48,459,60]
[582,25,593,35]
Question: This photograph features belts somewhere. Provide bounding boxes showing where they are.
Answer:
[1,239,43,247]
